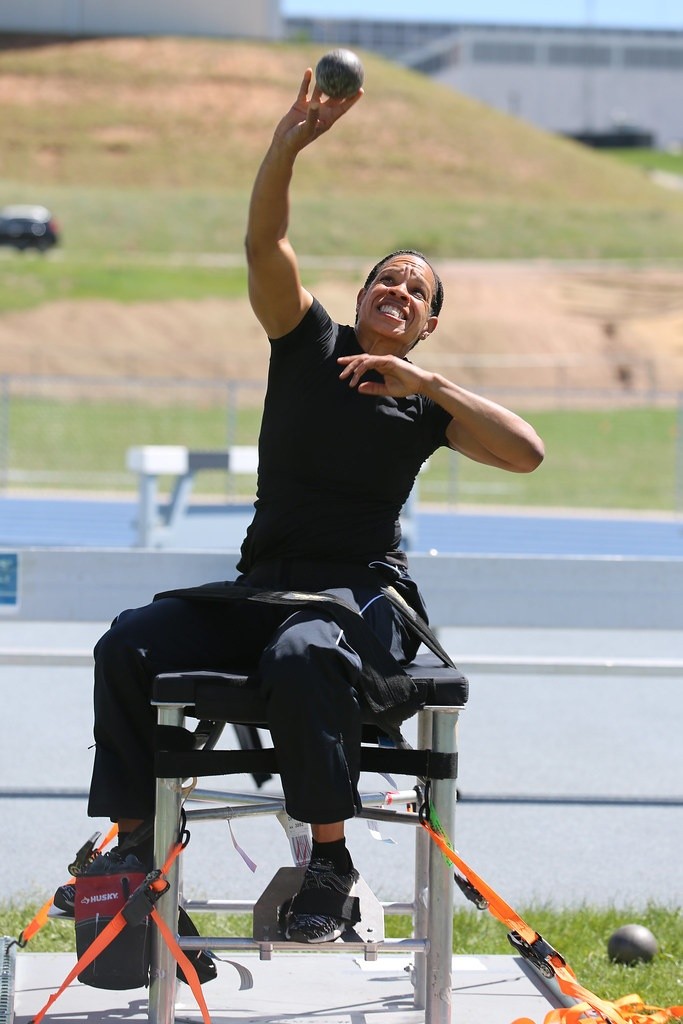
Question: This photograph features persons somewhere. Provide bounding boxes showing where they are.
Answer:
[55,66,545,943]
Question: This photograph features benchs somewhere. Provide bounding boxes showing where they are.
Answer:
[129,443,257,544]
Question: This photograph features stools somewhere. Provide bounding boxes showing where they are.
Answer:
[148,651,469,1024]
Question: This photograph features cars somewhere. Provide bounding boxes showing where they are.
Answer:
[0,206,58,253]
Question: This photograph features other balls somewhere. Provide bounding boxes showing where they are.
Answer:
[317,47,364,97]
[608,925,658,964]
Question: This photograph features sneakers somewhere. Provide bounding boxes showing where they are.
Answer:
[285,859,360,943]
[54,846,153,912]
